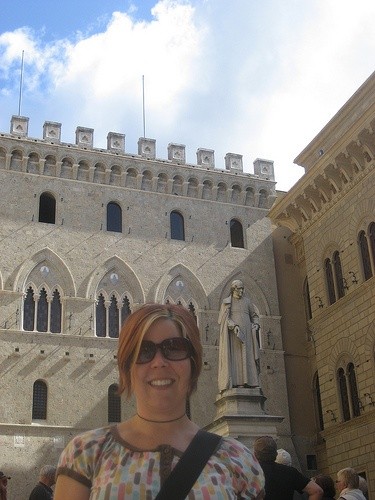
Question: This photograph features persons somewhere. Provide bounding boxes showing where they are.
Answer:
[253,435,324,500]
[31,464,58,500]
[336,467,368,500]
[307,473,336,499]
[217,278,263,393]
[53,304,267,500]
[274,448,293,466]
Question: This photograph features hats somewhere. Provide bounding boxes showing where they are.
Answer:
[0,472,11,483]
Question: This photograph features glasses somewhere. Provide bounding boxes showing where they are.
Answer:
[136,337,192,364]
[335,480,341,483]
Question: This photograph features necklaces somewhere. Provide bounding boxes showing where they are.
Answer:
[135,410,190,423]
